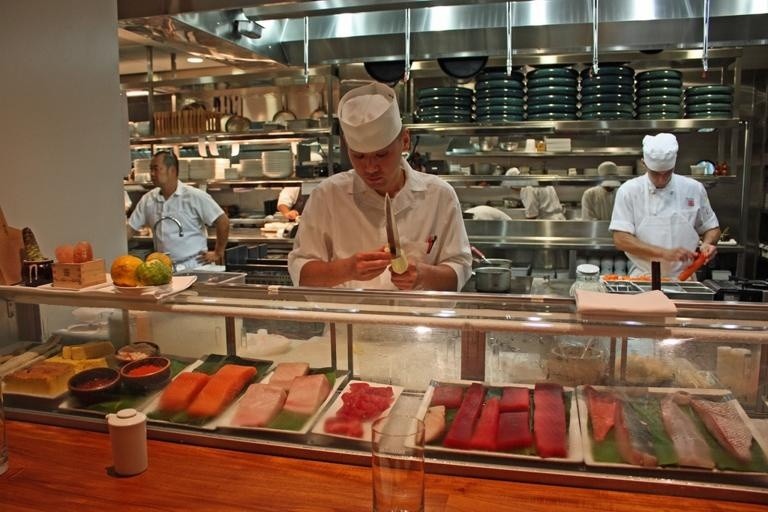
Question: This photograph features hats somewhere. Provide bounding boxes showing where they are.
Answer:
[336,81,402,155]
[504,167,520,189]
[597,161,621,188]
[642,133,679,172]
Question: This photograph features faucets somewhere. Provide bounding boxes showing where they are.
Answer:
[152,216,184,251]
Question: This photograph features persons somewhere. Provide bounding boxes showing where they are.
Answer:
[505,167,568,220]
[276,151,323,222]
[127,150,230,271]
[581,161,621,222]
[463,204,512,222]
[608,132,722,281]
[286,81,474,343]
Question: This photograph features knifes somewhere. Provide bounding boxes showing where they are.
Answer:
[383,191,408,275]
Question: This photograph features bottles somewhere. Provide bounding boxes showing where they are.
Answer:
[105,410,150,476]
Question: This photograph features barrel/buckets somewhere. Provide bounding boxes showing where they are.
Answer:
[549,346,606,386]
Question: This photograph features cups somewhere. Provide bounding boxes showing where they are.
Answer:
[370,412,427,512]
[524,139,538,152]
[690,166,706,175]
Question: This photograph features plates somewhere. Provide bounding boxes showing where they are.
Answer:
[474,67,526,121]
[545,138,572,152]
[547,166,634,175]
[526,67,579,121]
[636,69,685,119]
[684,84,734,120]
[131,151,296,179]
[580,66,636,119]
[417,86,474,122]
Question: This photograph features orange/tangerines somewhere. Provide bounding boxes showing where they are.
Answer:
[111,252,172,287]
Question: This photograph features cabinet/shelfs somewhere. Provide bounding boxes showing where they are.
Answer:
[125,49,754,276]
[0,277,767,499]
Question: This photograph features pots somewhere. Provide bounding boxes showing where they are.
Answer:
[364,60,414,87]
[438,55,486,80]
[271,89,330,123]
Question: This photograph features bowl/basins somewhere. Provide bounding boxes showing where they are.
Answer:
[68,339,171,404]
[476,266,511,291]
[551,345,605,383]
[498,142,518,151]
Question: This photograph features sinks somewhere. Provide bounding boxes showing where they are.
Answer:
[173,269,246,284]
